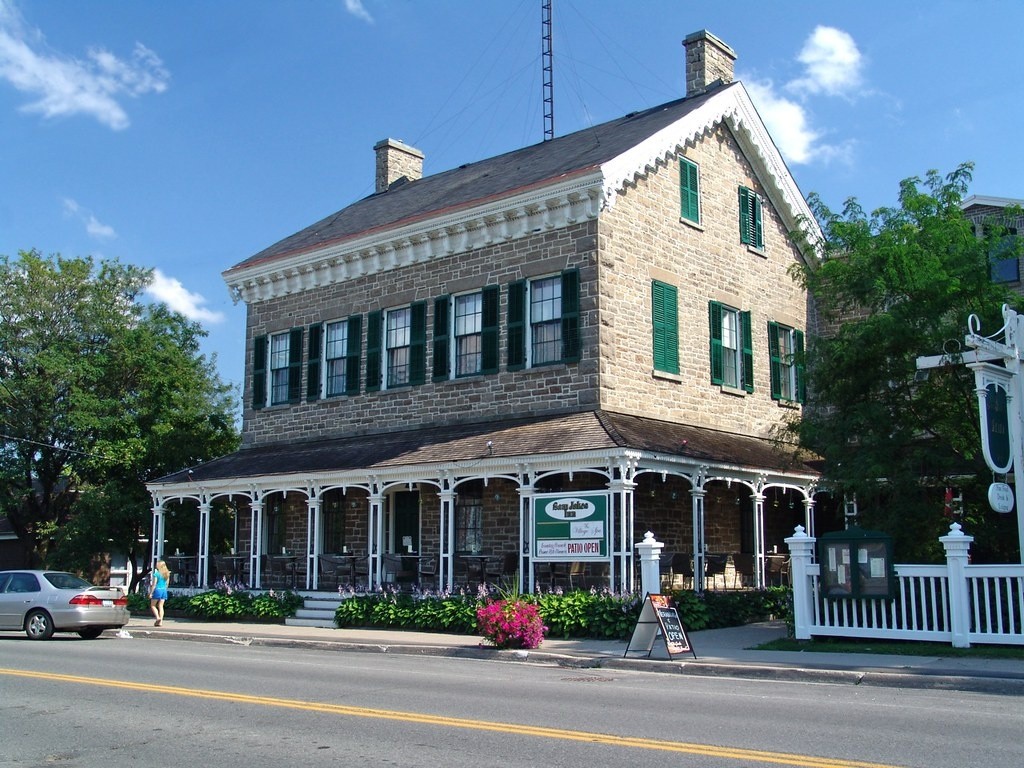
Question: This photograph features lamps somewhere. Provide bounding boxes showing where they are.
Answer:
[670,492,677,500]
[788,488,794,509]
[289,504,294,512]
[773,487,781,507]
[717,496,722,504]
[495,492,502,502]
[349,501,357,509]
[273,505,278,512]
[735,498,741,505]
[650,489,656,497]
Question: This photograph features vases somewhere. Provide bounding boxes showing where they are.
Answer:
[499,636,522,650]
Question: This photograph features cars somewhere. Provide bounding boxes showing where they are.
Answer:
[0,571,131,640]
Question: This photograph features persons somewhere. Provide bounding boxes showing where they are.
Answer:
[148,560,170,627]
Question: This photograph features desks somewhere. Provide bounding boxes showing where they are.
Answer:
[269,555,303,590]
[167,557,196,585]
[455,553,494,591]
[397,556,430,591]
[692,554,719,592]
[746,555,785,591]
[334,555,366,592]
[220,556,249,590]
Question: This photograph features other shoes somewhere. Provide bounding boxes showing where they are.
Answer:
[154,618,161,627]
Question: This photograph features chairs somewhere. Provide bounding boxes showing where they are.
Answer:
[170,551,793,593]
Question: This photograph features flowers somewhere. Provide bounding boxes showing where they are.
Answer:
[474,570,548,651]
